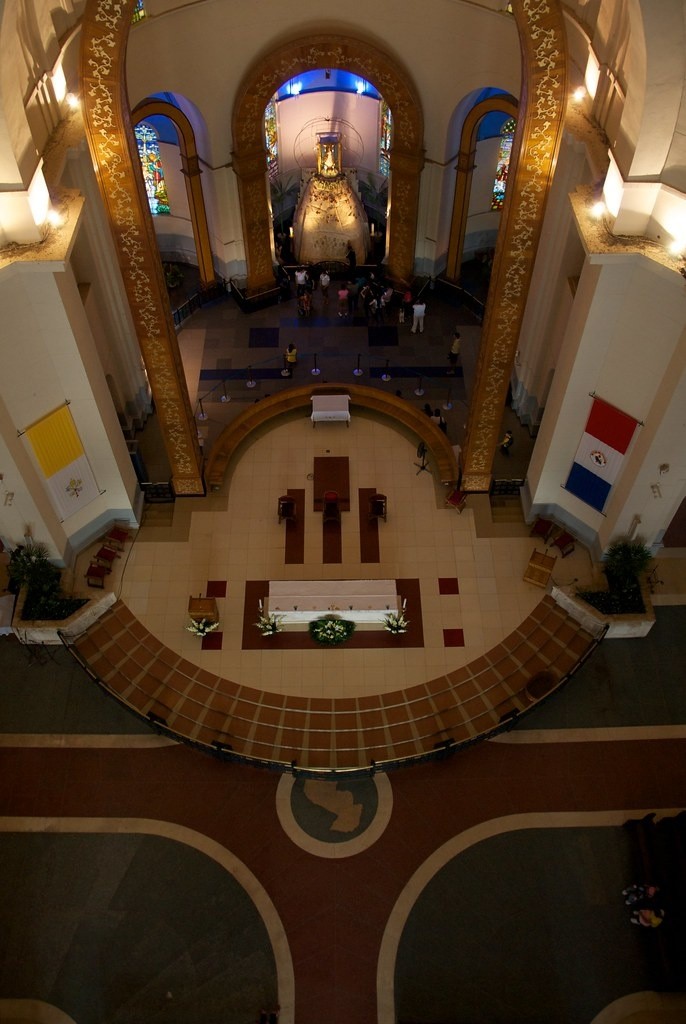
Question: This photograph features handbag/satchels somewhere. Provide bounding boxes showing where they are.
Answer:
[439,417,447,430]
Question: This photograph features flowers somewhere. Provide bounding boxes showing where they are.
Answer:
[379,613,411,636]
[185,617,221,638]
[252,613,289,636]
[309,619,356,646]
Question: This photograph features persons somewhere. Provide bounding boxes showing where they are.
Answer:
[621,884,660,905]
[431,409,446,426]
[337,284,349,317]
[345,246,356,284]
[285,344,297,380]
[403,290,412,305]
[319,270,330,305]
[412,298,427,334]
[369,271,376,281]
[630,908,665,927]
[259,1008,279,1024]
[395,390,406,400]
[361,285,394,314]
[447,332,460,375]
[398,305,406,323]
[295,267,315,318]
[497,431,513,459]
[422,403,433,417]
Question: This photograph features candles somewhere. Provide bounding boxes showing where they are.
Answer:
[403,598,407,608]
[259,599,263,610]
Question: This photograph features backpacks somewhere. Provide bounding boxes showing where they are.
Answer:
[506,437,513,446]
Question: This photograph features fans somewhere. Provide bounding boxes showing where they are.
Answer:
[414,442,433,475]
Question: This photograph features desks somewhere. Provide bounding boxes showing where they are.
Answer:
[268,581,398,623]
[309,388,352,428]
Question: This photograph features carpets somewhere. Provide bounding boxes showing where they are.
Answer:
[285,488,306,564]
[323,512,341,564]
[359,487,380,563]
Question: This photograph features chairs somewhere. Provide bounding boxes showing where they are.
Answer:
[528,512,557,545]
[548,527,576,559]
[322,490,340,513]
[367,493,388,523]
[277,495,297,525]
[103,519,133,552]
[83,560,111,590]
[443,488,470,515]
[92,543,121,573]
